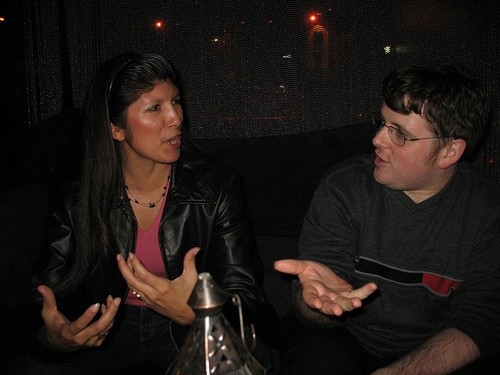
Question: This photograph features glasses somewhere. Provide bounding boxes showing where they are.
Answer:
[372,110,453,147]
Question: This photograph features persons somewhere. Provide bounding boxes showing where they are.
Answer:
[38,53,262,375]
[274,59,500,375]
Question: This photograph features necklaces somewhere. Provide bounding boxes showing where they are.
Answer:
[123,173,170,208]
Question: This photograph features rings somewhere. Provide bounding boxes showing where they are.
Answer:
[98,334,106,339]
[133,290,141,298]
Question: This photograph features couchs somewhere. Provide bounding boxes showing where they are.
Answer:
[0,127,370,375]
